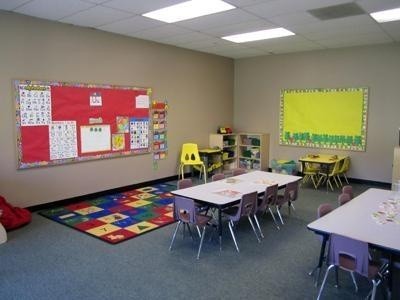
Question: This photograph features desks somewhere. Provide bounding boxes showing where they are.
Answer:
[299,156,345,192]
[196,149,221,179]
[307,187,400,287]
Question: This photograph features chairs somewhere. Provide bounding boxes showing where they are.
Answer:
[343,186,353,200]
[299,156,319,191]
[317,203,334,216]
[328,157,350,185]
[177,143,207,183]
[306,154,320,182]
[317,233,391,300]
[319,159,343,192]
[168,170,303,259]
[338,194,350,206]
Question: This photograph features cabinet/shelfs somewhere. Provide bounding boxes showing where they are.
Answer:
[391,147,400,191]
[209,132,269,172]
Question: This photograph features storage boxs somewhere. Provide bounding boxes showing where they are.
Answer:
[273,163,300,176]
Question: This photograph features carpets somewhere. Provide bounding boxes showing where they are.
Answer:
[38,182,178,245]
